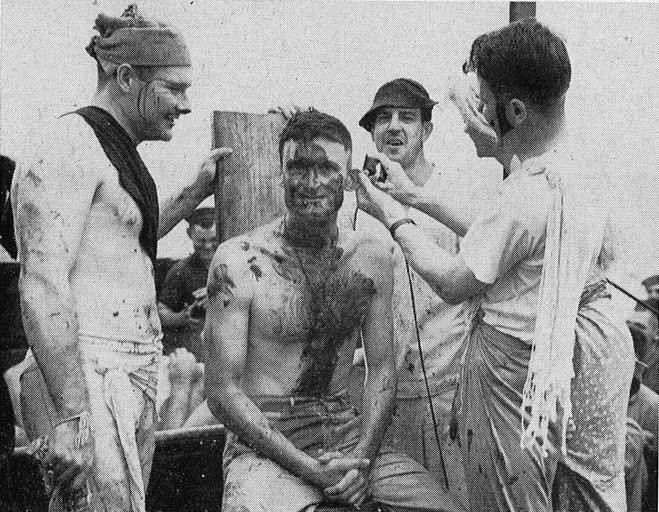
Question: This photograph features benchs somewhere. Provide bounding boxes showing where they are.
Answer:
[0,422,382,512]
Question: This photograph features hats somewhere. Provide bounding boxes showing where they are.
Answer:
[358,75,440,132]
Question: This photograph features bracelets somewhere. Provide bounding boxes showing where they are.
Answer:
[390,218,416,240]
[53,412,93,427]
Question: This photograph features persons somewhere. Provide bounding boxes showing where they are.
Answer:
[449,71,636,511]
[268,78,485,512]
[157,347,223,431]
[622,275,659,512]
[355,16,605,512]
[9,3,232,512]
[202,109,472,512]
[153,208,226,352]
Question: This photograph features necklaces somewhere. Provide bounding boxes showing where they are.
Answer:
[284,216,339,314]
